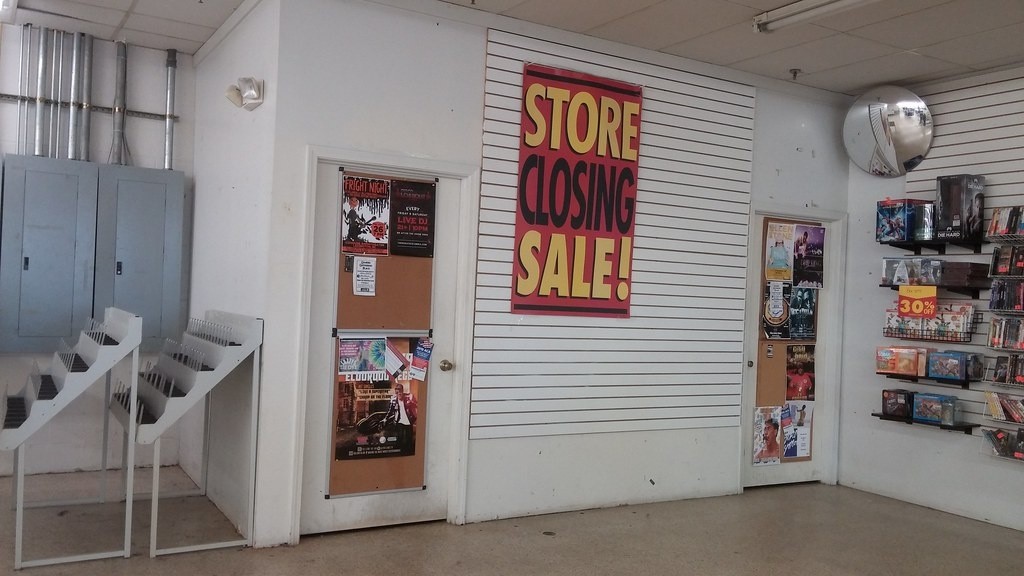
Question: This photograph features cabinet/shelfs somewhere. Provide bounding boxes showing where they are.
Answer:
[977,234,1024,464]
[871,238,993,436]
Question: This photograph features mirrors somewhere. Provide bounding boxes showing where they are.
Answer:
[841,85,935,179]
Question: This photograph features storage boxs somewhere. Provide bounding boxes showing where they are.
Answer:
[882,256,934,286]
[875,343,965,423]
[936,174,988,237]
[875,198,935,242]
[920,260,992,286]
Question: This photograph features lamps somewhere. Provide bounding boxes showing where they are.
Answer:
[225,72,265,112]
